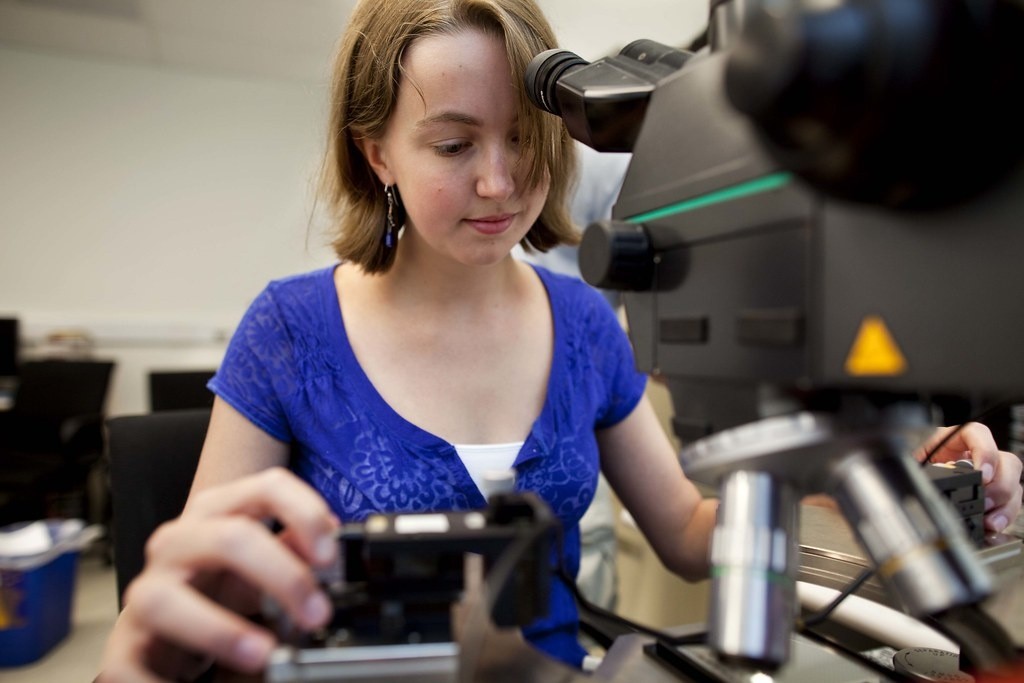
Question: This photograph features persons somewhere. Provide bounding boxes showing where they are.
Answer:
[99,0,1022,683]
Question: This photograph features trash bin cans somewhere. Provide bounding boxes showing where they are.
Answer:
[0,518,103,669]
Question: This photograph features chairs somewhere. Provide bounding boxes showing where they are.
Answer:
[0,357,115,525]
[100,410,213,616]
